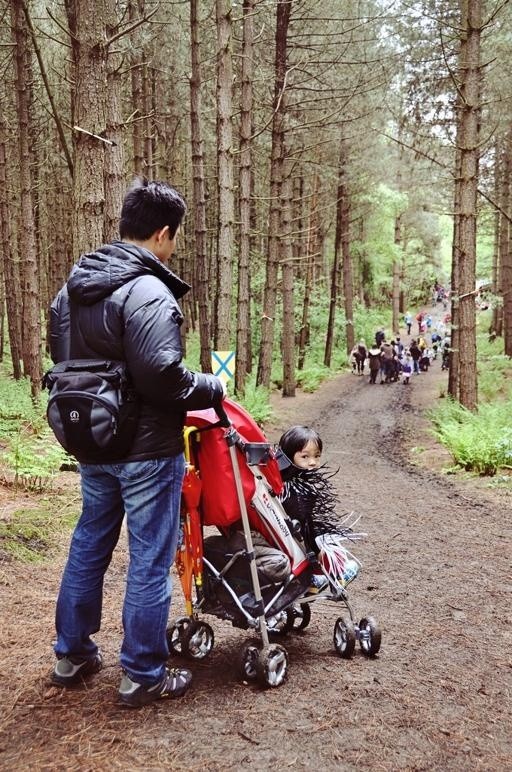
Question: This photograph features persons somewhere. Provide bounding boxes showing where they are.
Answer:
[351,277,452,387]
[46,179,226,711]
[274,424,360,596]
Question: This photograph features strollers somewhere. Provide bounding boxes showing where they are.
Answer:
[161,396,385,689]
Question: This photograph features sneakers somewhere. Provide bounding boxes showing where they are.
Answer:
[117,664,192,709]
[50,652,102,687]
[310,559,359,594]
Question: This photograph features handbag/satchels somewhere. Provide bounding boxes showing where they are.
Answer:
[41,359,141,466]
